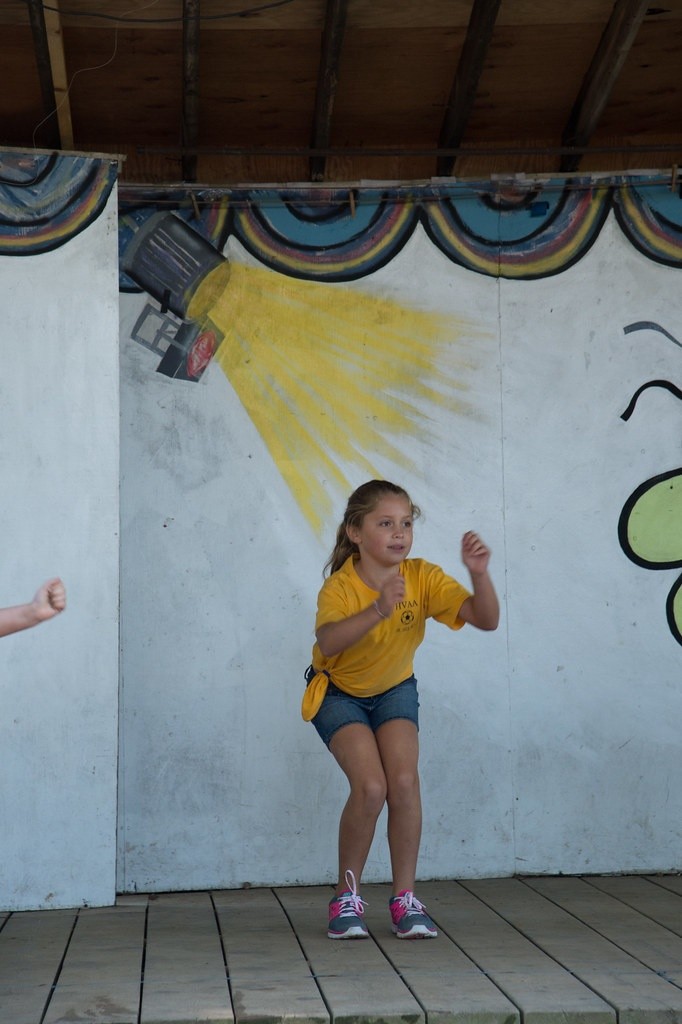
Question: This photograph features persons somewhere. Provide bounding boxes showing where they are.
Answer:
[0,577,67,639]
[301,480,500,940]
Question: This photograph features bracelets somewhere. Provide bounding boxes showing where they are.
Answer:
[373,600,385,617]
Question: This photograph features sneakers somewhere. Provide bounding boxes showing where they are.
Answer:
[328,869,370,939]
[389,890,437,940]
